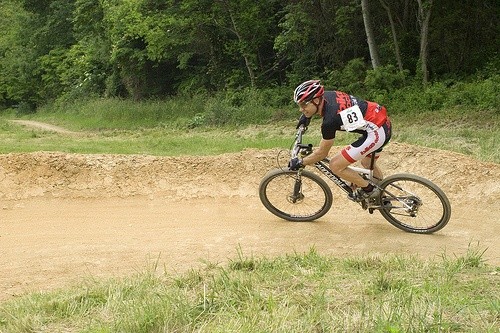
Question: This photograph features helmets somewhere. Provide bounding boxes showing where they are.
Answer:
[293,78,324,105]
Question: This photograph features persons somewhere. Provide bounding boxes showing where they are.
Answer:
[289,79,391,199]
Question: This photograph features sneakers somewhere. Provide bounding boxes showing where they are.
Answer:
[347,185,380,201]
[382,195,392,212]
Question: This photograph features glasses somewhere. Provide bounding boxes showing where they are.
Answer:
[299,98,313,108]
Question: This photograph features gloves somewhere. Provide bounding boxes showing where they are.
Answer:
[296,113,311,129]
[290,157,305,171]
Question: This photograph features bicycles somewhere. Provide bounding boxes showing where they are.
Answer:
[257,121,452,235]
